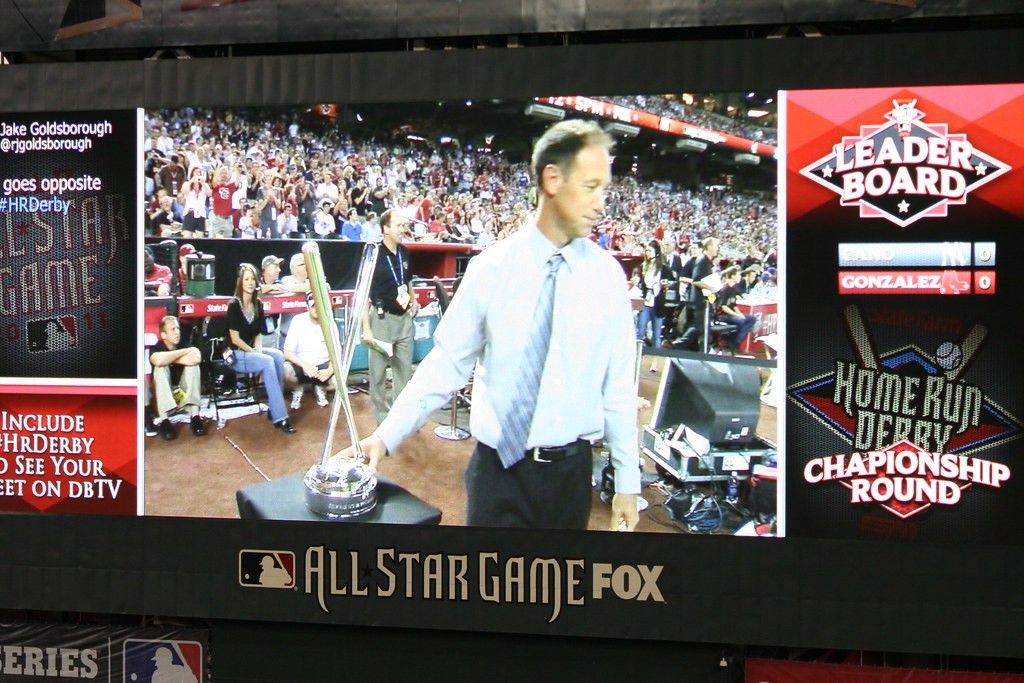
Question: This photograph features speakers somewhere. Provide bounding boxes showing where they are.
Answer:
[648,357,760,442]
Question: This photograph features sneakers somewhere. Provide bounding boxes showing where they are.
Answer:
[145,422,157,436]
[158,418,174,439]
[190,415,204,435]
[291,390,303,409]
[312,384,329,406]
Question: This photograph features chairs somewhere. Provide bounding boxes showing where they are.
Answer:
[142,96,779,441]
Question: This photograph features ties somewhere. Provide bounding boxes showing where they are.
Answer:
[496,254,564,467]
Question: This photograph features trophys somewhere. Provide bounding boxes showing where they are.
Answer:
[301,241,379,519]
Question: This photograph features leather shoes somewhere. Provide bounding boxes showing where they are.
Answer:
[275,418,296,432]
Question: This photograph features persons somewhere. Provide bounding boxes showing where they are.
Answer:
[142,97,777,258]
[361,208,419,427]
[671,237,720,353]
[281,252,331,291]
[712,259,778,354]
[148,315,207,441]
[636,239,676,373]
[282,290,342,408]
[178,244,200,293]
[334,117,644,533]
[145,246,173,296]
[221,262,296,433]
[661,237,684,339]
[260,254,288,294]
[679,240,704,302]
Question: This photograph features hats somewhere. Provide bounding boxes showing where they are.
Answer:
[306,290,315,308]
